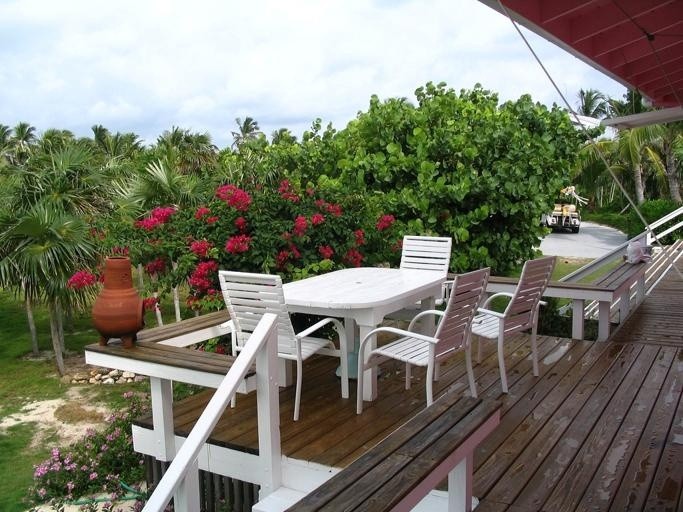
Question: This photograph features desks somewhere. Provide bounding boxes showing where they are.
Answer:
[254,268,447,400]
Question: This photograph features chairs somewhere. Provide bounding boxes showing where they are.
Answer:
[383,234,454,337]
[216,266,347,422]
[355,265,491,412]
[445,254,559,393]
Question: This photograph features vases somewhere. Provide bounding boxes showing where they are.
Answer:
[91,255,147,347]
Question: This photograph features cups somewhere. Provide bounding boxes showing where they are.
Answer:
[627,242,641,264]
[642,246,652,258]
